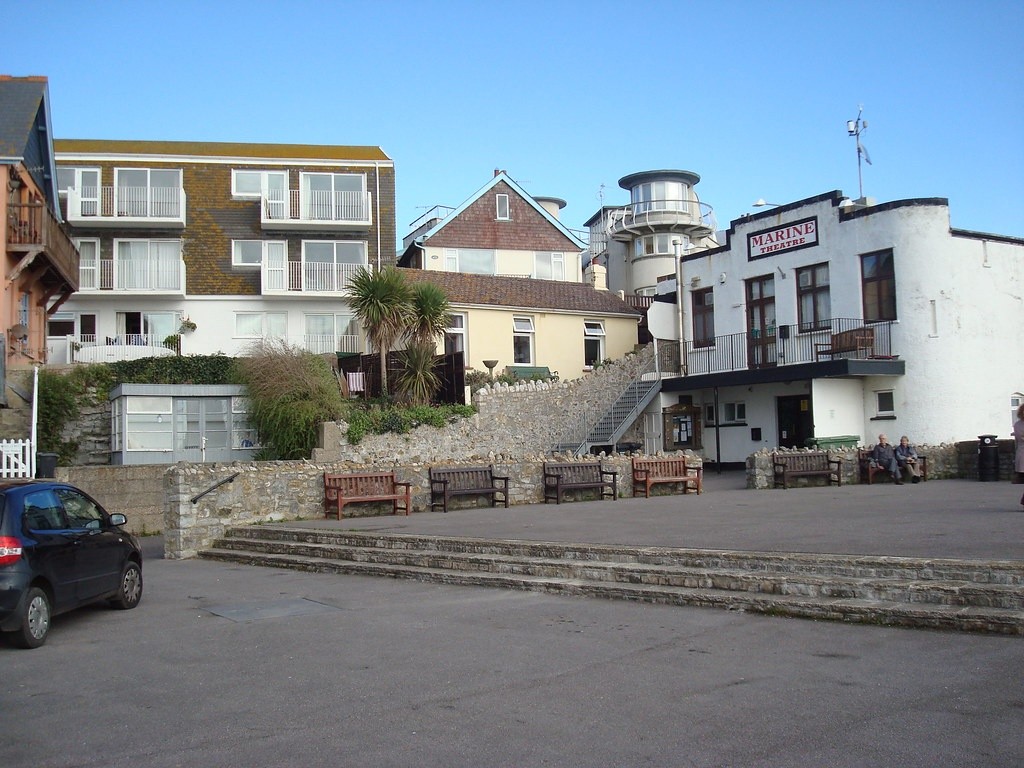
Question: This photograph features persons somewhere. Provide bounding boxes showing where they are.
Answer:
[872,434,904,485]
[1014,404,1024,505]
[896,436,921,484]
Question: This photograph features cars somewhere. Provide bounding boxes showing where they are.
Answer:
[0,481,143,649]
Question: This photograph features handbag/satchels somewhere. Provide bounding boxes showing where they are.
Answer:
[1010,473,1024,484]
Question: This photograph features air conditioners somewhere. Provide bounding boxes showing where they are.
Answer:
[799,272,811,287]
[705,293,714,306]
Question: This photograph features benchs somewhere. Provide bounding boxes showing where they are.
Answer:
[323,469,410,521]
[506,365,559,383]
[543,460,618,504]
[814,328,875,363]
[859,448,927,485]
[429,464,510,513]
[773,450,841,490]
[632,456,703,499]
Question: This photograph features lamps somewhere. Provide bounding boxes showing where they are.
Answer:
[752,199,780,208]
[422,235,431,243]
[839,199,867,208]
[9,180,20,193]
[11,324,27,359]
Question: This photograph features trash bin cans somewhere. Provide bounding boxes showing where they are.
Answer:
[977,435,1000,481]
[38,452,60,478]
[804,435,861,450]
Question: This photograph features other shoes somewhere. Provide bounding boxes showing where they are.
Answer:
[896,479,904,485]
[912,476,920,483]
[889,472,895,480]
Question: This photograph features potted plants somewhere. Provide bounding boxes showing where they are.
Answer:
[28,370,81,479]
